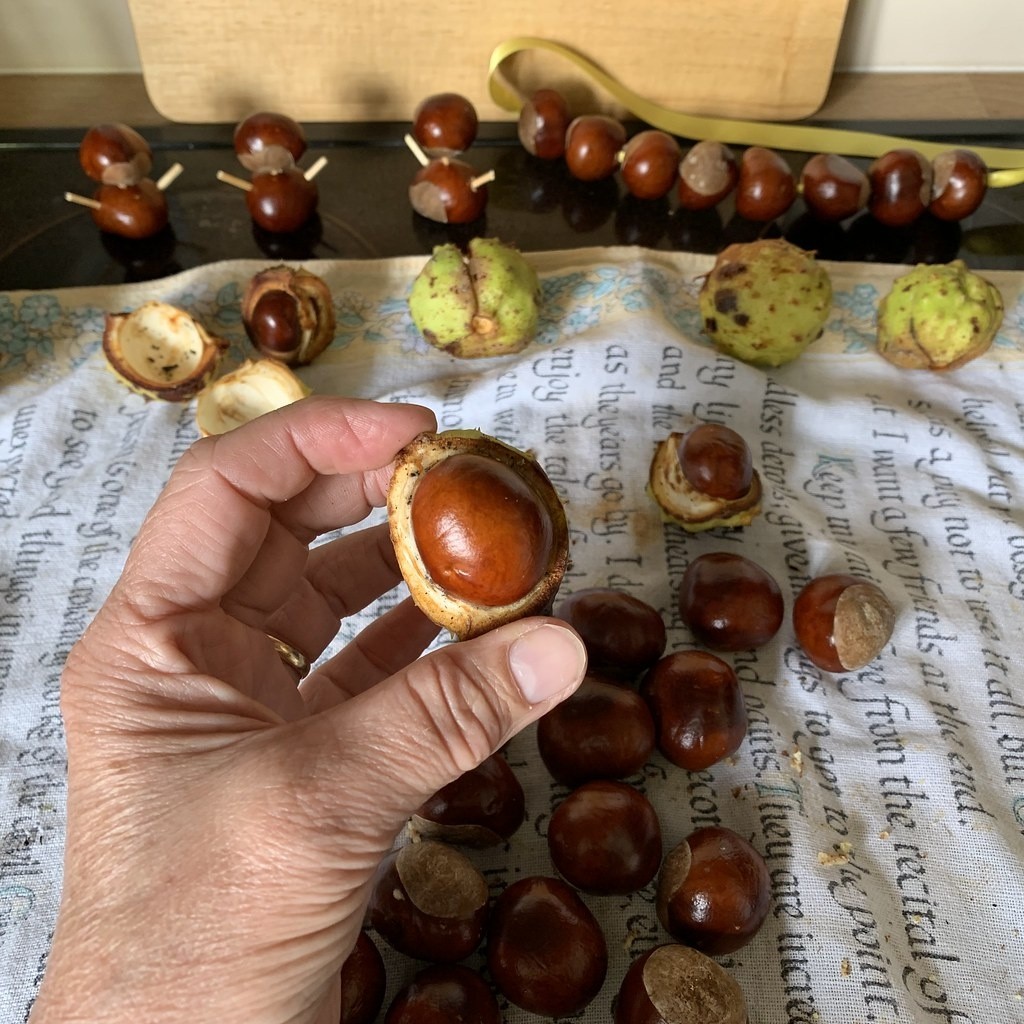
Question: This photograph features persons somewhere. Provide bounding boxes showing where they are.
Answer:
[25,398,592,1023]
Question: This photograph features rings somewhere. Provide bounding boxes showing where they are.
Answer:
[265,631,311,679]
